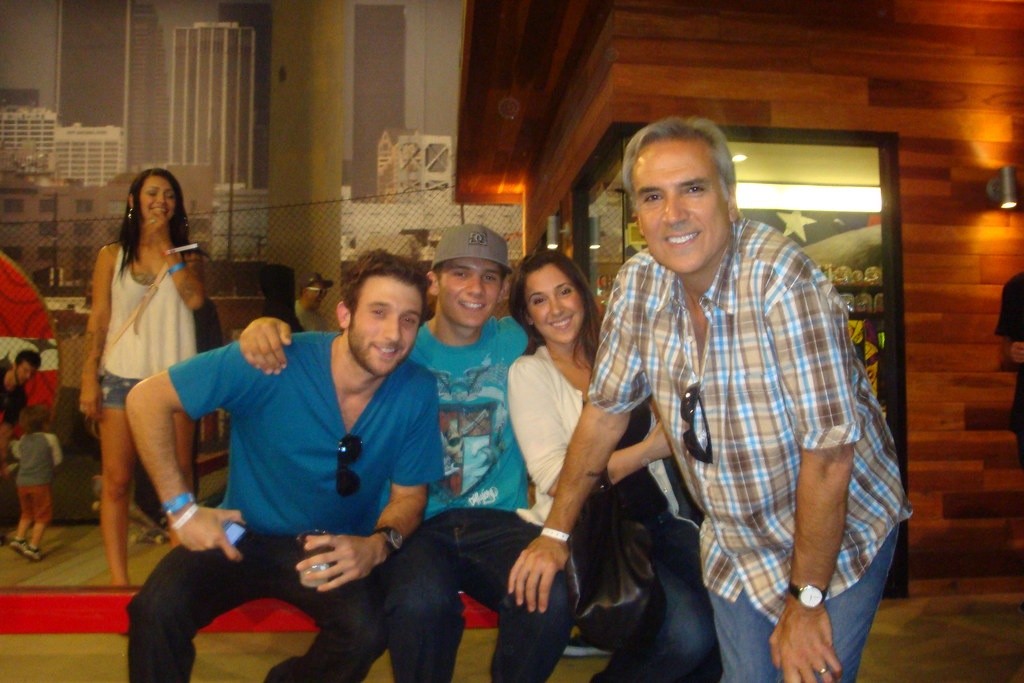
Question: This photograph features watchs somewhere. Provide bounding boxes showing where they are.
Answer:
[788,581,828,607]
[372,526,403,553]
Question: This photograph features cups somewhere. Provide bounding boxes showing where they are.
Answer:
[296,530,334,592]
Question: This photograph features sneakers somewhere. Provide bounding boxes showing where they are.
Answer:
[11,536,24,554]
[21,545,41,561]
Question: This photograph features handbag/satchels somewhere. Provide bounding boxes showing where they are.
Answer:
[85,380,100,437]
[566,473,662,653]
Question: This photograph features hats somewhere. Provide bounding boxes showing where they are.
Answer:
[429,224,512,275]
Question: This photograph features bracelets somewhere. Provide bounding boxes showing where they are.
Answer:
[540,528,569,541]
[169,503,197,531]
[167,261,185,275]
[163,243,199,256]
[162,493,194,518]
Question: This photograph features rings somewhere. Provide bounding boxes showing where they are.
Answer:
[819,666,830,675]
[148,220,156,224]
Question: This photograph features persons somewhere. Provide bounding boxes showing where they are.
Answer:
[994,272,1024,613]
[78,167,206,588]
[126,248,446,683]
[239,224,574,683]
[508,247,723,683]
[509,118,912,683]
[293,273,336,333]
[0,351,64,560]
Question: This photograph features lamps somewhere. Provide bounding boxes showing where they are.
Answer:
[986,165,1018,210]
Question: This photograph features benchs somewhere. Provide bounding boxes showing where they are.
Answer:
[0,586,499,635]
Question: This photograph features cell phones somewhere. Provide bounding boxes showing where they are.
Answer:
[222,520,247,548]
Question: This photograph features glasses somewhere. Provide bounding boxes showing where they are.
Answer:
[336,434,361,497]
[680,383,714,463]
